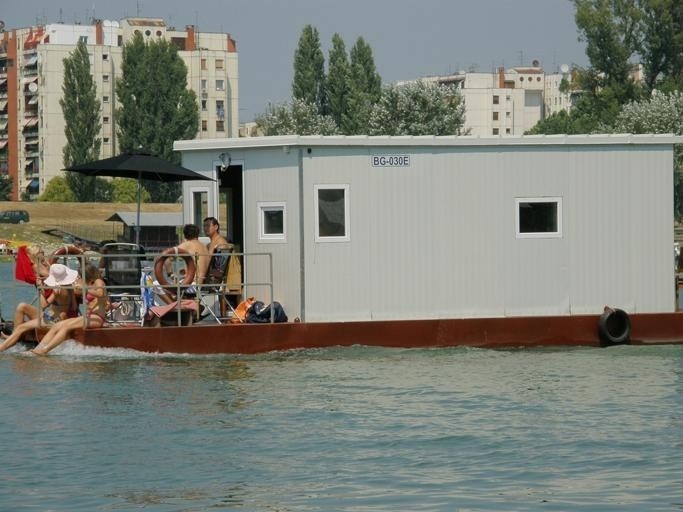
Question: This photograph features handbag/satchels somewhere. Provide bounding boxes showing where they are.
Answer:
[230,297,288,325]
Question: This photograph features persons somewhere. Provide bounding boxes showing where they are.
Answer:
[203,217,227,264]
[153,273,176,306]
[0,263,78,352]
[173,224,209,320]
[27,245,122,312]
[31,264,107,355]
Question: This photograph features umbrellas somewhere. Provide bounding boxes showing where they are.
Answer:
[60,145,217,245]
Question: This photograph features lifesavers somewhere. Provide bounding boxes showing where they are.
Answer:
[50,246,85,268]
[154,248,194,291]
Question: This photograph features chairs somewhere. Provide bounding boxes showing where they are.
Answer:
[105,242,143,322]
[174,243,244,326]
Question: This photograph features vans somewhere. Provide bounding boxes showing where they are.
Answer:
[0,210,29,224]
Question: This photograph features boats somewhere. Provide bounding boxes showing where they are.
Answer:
[1,134,682,357]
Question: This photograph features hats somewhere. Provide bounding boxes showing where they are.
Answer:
[43,263,78,287]
